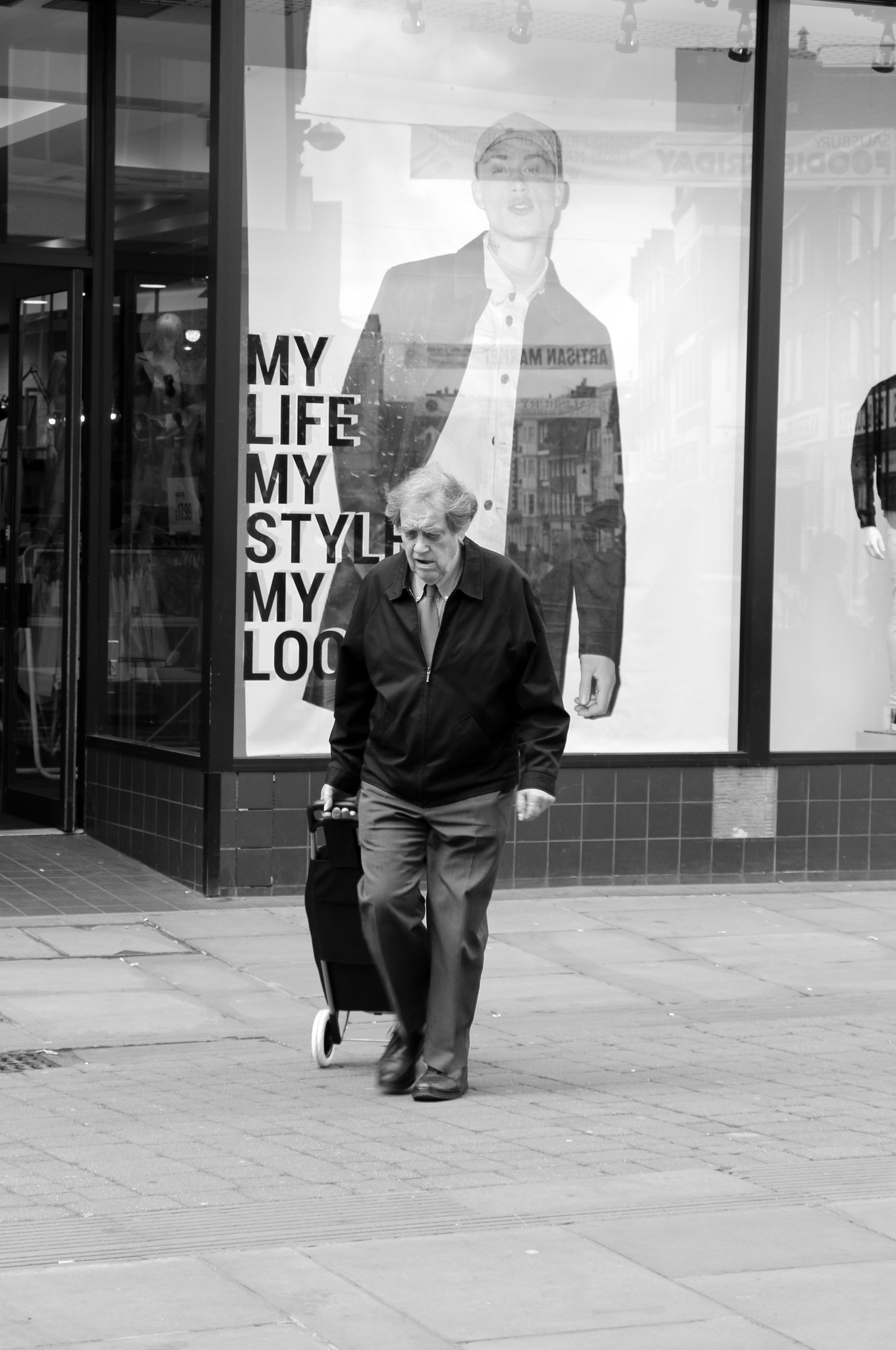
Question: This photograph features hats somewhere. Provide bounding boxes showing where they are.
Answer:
[473,113,560,181]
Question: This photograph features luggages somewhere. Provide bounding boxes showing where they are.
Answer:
[303,800,395,1067]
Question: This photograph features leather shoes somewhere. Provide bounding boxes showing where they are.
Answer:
[411,1066,469,1100]
[380,1025,425,1090]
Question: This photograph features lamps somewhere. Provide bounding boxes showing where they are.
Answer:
[728,12,753,62]
[508,0,534,44]
[872,26,896,73]
[615,2,638,53]
[401,0,425,35]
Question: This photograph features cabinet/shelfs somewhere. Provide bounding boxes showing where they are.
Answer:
[30,547,204,750]
[851,376,896,527]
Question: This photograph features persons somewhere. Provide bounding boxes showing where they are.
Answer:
[301,111,627,722]
[119,310,204,549]
[852,374,896,742]
[321,467,573,1103]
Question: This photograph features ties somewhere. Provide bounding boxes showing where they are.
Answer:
[420,586,440,670]
